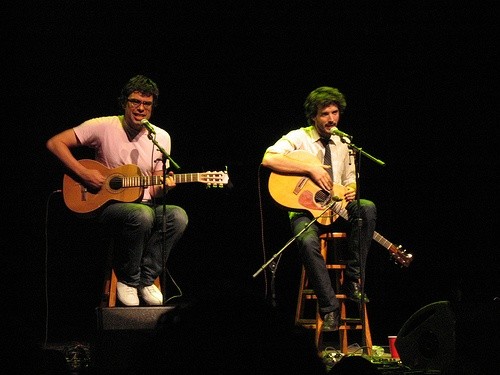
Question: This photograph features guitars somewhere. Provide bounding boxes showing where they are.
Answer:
[267,149,414,270]
[64,158,230,214]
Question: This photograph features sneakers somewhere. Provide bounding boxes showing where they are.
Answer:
[139,283,164,306]
[116,281,140,307]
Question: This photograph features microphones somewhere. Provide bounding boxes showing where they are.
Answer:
[330,126,349,137]
[141,119,157,135]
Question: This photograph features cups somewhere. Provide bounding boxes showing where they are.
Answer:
[388,336,399,358]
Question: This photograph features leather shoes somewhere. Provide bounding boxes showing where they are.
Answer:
[340,280,370,303]
[321,312,341,332]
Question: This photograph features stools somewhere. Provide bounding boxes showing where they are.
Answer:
[104,234,160,308]
[296,232,372,358]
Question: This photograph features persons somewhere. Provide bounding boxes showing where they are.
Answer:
[1,291,500,375]
[45,74,188,307]
[262,86,377,331]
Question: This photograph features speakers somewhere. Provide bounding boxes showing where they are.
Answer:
[90,307,176,358]
[394,299,500,370]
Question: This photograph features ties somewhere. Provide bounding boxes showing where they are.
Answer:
[319,138,334,182]
